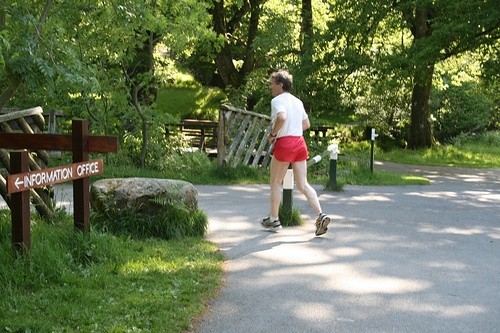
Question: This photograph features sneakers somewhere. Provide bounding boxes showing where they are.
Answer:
[315,213,330,236]
[259,217,282,231]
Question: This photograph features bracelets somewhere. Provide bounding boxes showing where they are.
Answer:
[270,132,277,137]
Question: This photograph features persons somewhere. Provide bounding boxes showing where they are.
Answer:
[259,69,331,236]
